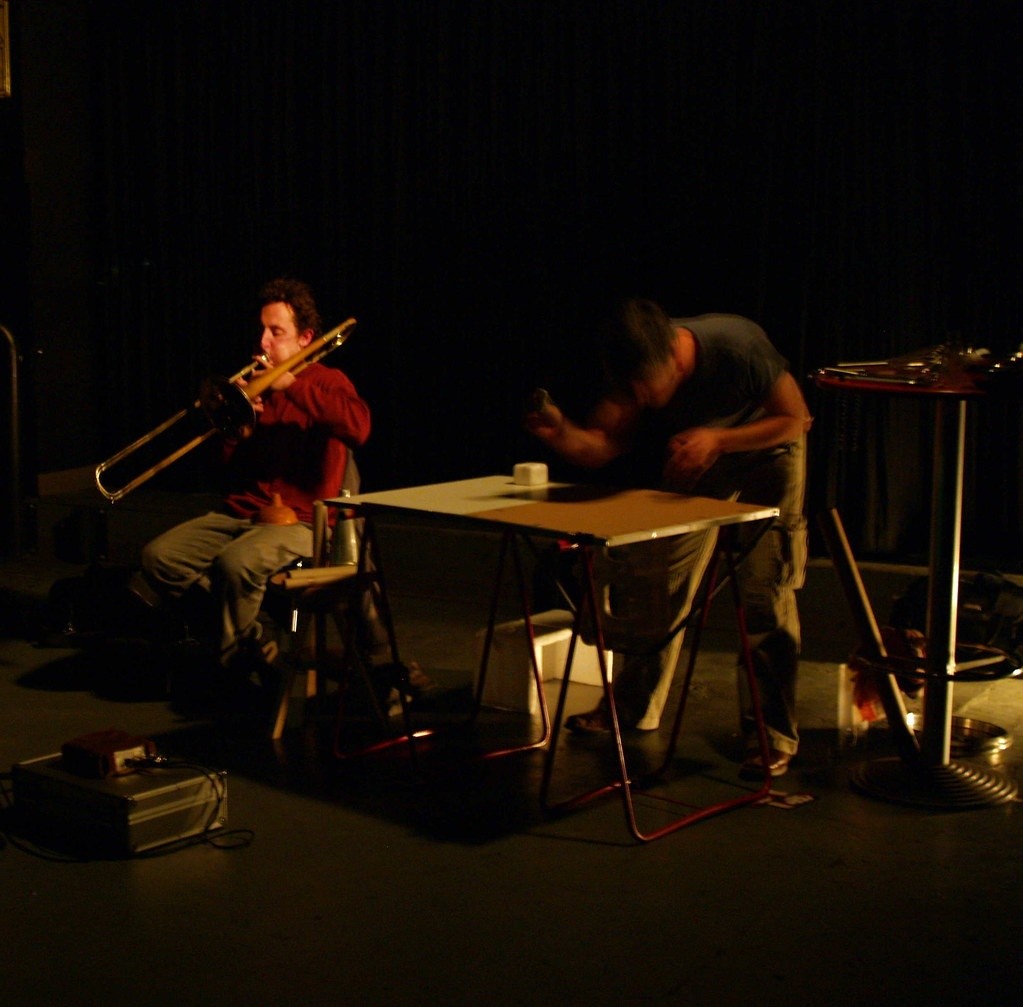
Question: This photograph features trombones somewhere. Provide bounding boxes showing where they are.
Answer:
[90,322,341,506]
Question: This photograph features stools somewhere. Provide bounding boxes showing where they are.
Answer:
[473,608,616,715]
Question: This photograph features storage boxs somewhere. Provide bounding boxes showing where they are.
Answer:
[13,751,227,851]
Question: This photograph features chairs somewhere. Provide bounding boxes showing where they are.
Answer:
[263,466,403,735]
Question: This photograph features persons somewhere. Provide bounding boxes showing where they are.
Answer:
[141,277,372,717]
[527,296,810,773]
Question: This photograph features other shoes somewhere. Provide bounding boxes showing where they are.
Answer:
[567,708,639,734]
[240,628,281,674]
[740,745,796,778]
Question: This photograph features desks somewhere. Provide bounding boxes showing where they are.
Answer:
[819,352,1023,809]
[321,473,780,836]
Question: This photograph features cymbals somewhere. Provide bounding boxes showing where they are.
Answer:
[809,345,1021,399]
